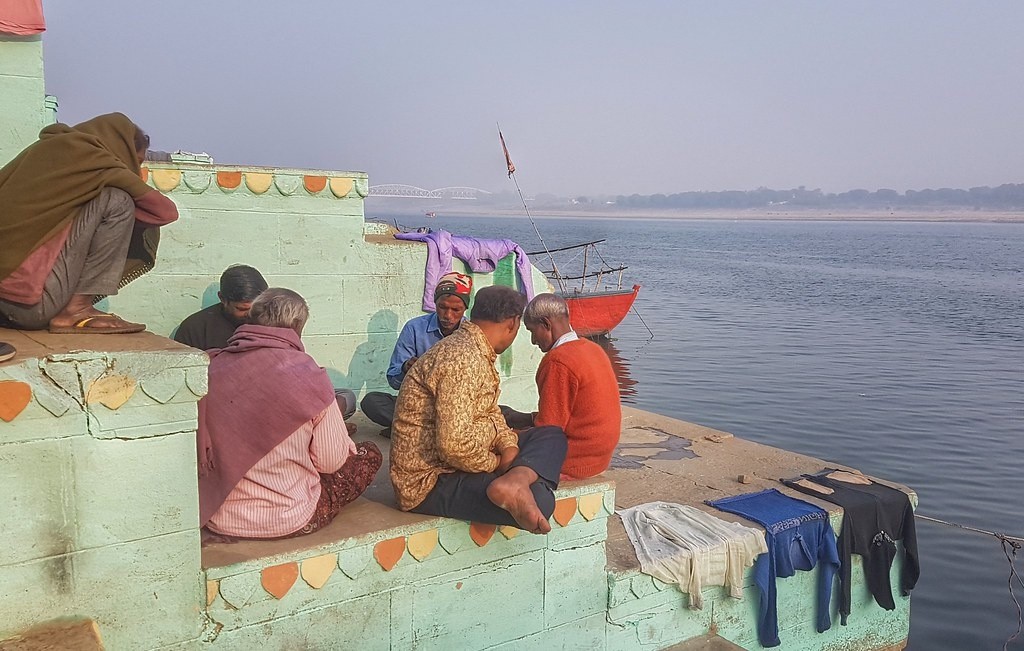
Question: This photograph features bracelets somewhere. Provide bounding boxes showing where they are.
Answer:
[406,361,408,370]
[531,413,533,423]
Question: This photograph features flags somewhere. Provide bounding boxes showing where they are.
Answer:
[499,131,516,176]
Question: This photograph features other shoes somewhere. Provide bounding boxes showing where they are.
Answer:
[0,342,17,361]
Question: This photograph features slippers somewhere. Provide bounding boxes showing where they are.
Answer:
[49,312,146,333]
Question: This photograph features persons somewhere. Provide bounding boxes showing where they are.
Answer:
[390,285,568,535]
[175,263,358,436]
[0,112,179,335]
[196,288,384,548]
[361,271,474,426]
[503,292,621,478]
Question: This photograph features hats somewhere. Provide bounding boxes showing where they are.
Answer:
[433,272,473,310]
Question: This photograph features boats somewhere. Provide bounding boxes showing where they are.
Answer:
[524,238,641,339]
